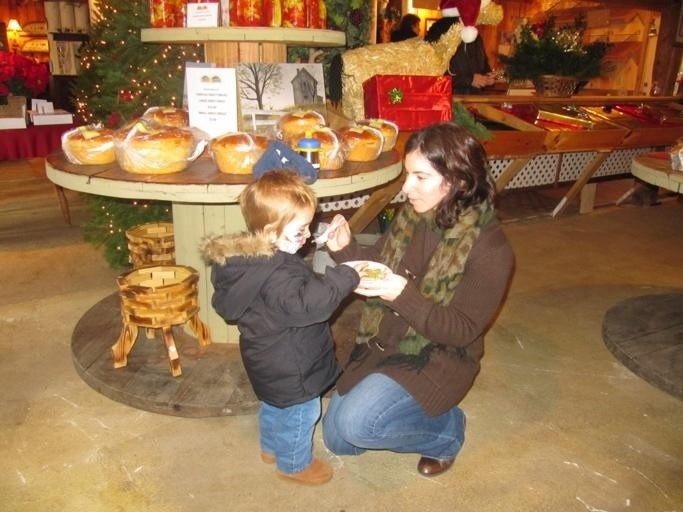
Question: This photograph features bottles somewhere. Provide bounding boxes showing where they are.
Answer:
[299,138,319,174]
[649,81,661,96]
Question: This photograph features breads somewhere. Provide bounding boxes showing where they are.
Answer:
[356,119,398,152]
[286,122,348,170]
[335,127,384,162]
[142,105,189,125]
[59,121,118,164]
[114,119,194,173]
[273,109,324,142]
[209,131,273,175]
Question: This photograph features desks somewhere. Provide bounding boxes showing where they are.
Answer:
[44,111,408,422]
[601,149,683,399]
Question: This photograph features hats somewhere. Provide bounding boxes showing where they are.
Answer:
[252,141,318,185]
[439,0,484,45]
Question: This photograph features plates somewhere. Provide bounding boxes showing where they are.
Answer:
[339,261,393,288]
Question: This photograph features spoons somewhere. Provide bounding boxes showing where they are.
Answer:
[316,220,342,245]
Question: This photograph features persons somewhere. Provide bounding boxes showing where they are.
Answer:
[425,16,495,94]
[202,170,370,483]
[389,14,420,41]
[323,121,516,478]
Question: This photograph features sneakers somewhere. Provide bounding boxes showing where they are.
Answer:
[275,459,334,484]
[258,449,276,464]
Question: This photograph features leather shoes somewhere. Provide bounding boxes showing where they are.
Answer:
[417,412,467,477]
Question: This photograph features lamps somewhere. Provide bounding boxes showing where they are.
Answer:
[6,17,24,46]
